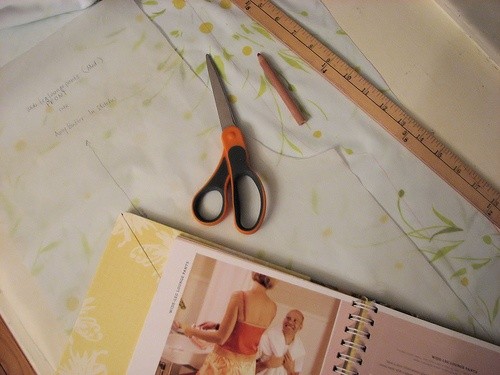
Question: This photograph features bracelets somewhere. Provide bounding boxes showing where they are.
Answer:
[215,323,220,330]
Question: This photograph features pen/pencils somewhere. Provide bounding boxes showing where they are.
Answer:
[256,52,306,127]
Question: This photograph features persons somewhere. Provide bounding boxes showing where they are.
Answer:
[256,309,306,375]
[184,271,277,375]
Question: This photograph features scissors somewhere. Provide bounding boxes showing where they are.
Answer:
[190,53,268,236]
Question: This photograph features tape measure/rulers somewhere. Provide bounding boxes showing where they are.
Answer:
[232,0,500,229]
